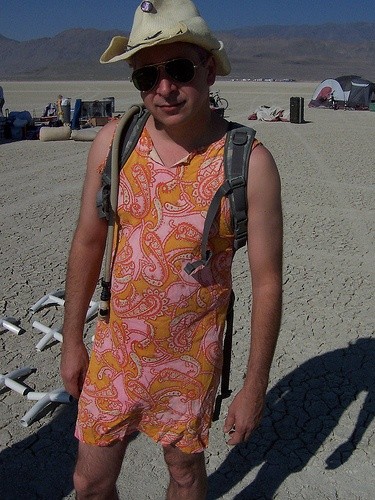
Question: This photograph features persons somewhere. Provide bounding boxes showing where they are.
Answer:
[59,0,284,500]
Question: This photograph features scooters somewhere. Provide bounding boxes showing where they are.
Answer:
[327,90,339,111]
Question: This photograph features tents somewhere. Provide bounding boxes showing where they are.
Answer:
[309,74,375,108]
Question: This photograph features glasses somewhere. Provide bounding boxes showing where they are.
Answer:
[128,57,206,93]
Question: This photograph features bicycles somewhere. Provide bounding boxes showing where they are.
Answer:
[210,90,228,110]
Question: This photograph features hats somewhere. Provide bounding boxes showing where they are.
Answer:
[99,0,231,76]
